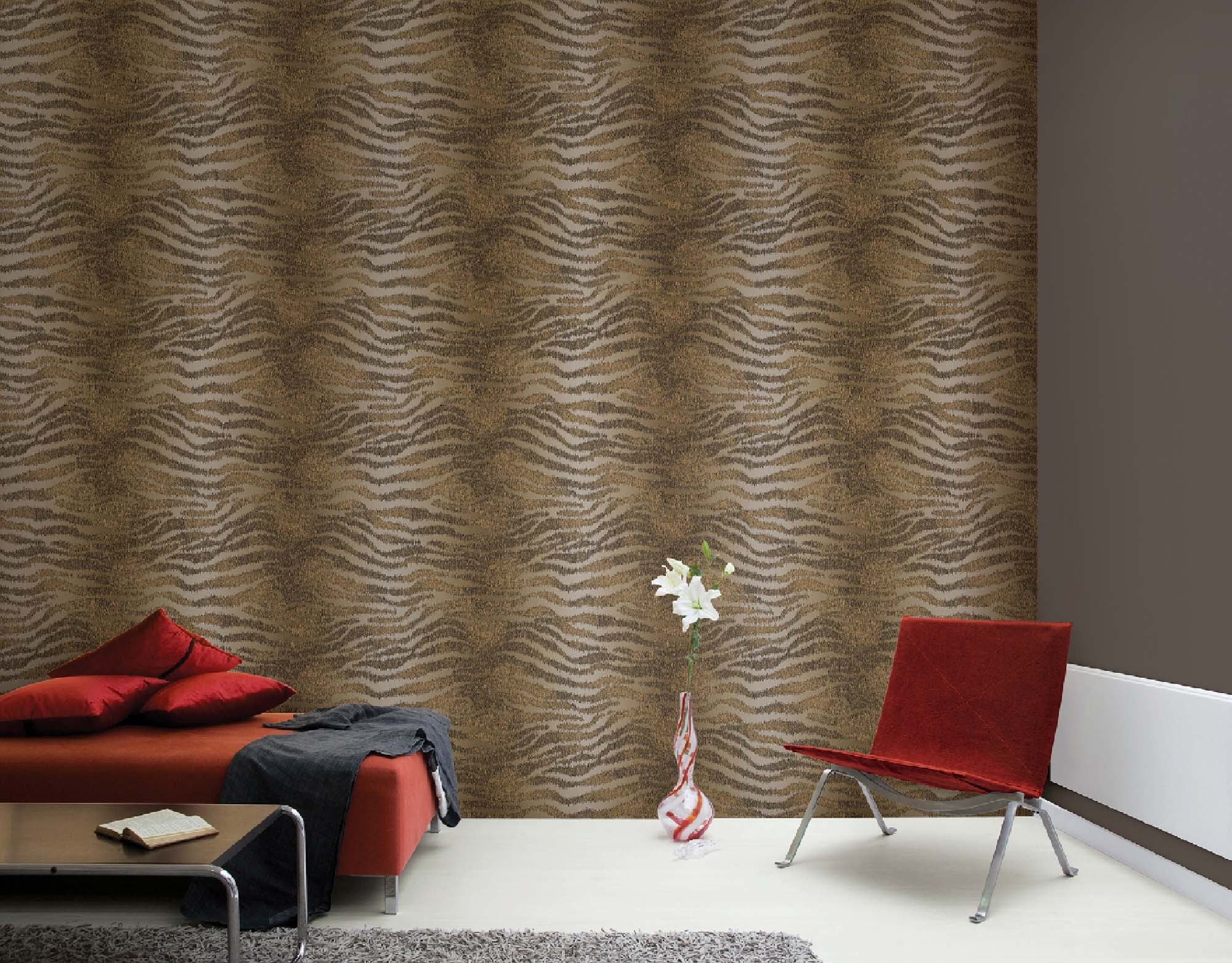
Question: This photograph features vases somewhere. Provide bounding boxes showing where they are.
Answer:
[657,691,715,843]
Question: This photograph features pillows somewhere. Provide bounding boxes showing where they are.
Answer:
[47,607,243,682]
[139,671,299,729]
[0,674,172,736]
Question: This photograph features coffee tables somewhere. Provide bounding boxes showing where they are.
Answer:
[0,802,308,963]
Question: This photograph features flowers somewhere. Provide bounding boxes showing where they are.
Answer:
[651,541,735,693]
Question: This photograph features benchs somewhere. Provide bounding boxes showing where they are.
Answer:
[0,714,442,916]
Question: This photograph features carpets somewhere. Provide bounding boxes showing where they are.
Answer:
[0,924,819,962]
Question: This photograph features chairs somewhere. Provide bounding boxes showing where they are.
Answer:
[774,616,1079,923]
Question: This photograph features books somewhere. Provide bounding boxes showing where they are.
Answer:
[94,807,218,850]
